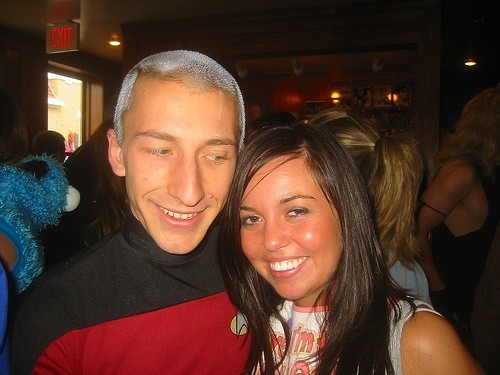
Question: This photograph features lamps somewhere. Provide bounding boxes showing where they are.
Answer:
[464,55,476,66]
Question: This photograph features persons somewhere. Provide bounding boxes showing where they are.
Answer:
[27,117,133,266]
[218,85,500,375]
[8,49,246,375]
[0,73,67,375]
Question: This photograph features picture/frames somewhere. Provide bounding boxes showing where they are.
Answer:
[372,84,393,108]
[303,99,327,118]
